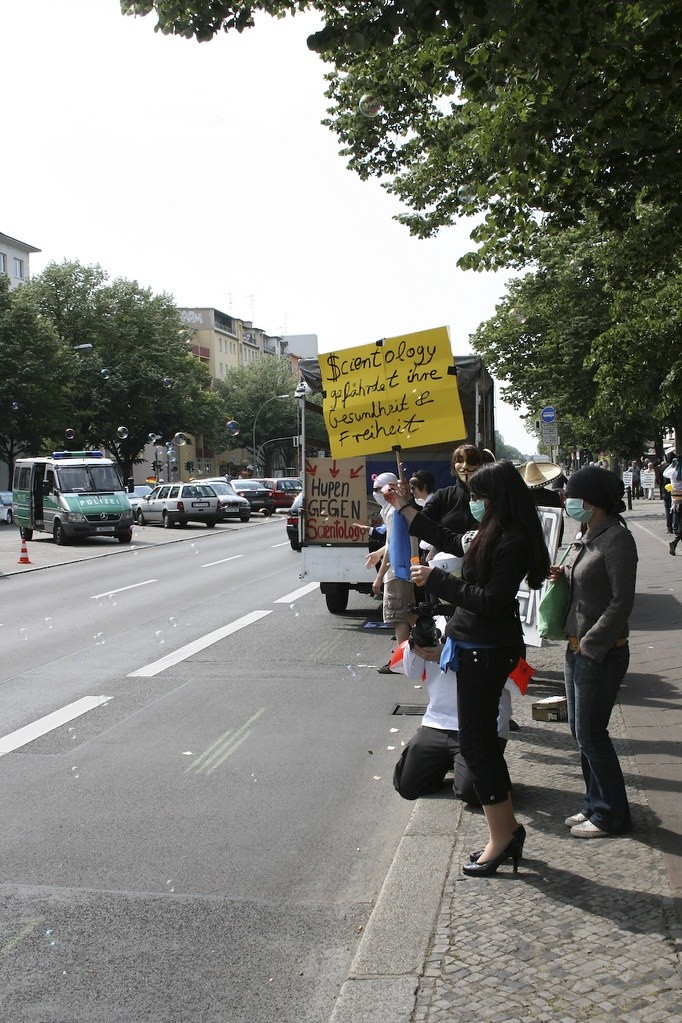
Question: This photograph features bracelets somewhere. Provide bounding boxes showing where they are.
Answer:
[397,503,411,514]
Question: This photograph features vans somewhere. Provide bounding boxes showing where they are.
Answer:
[12,451,134,545]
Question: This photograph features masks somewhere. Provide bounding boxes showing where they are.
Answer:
[565,498,594,523]
[469,500,487,522]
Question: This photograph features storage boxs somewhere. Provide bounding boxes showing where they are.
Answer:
[532,696,567,722]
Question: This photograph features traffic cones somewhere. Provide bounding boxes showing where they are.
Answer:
[17,539,31,564]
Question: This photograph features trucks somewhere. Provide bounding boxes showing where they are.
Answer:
[294,355,496,613]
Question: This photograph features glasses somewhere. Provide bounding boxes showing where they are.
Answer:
[411,487,416,490]
[470,493,478,500]
[374,488,382,492]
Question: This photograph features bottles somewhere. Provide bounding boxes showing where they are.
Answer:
[410,557,423,586]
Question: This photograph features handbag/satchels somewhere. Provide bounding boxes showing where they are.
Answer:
[539,573,570,641]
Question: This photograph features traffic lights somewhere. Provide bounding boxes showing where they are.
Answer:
[151,463,153,470]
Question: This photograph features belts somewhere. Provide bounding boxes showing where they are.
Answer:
[569,636,627,648]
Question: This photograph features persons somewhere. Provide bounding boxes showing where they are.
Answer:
[581,458,628,472]
[626,457,655,500]
[549,467,637,840]
[660,452,682,556]
[565,456,571,476]
[353,444,551,876]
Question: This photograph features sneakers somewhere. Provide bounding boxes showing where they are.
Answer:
[565,813,608,837]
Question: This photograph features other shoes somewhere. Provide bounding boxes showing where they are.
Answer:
[669,541,677,556]
[377,660,400,674]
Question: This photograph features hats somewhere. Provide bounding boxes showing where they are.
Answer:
[374,473,398,488]
[565,466,626,513]
[413,470,435,492]
[517,461,562,489]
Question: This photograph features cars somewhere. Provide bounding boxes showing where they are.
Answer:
[125,486,153,521]
[134,483,220,528]
[189,477,303,522]
[0,491,13,524]
[286,489,302,550]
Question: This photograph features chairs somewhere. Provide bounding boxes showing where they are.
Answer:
[172,492,178,497]
[184,492,192,498]
[194,492,202,498]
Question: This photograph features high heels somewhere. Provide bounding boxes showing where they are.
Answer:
[463,823,526,874]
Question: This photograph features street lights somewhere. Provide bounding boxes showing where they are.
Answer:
[253,394,290,477]
